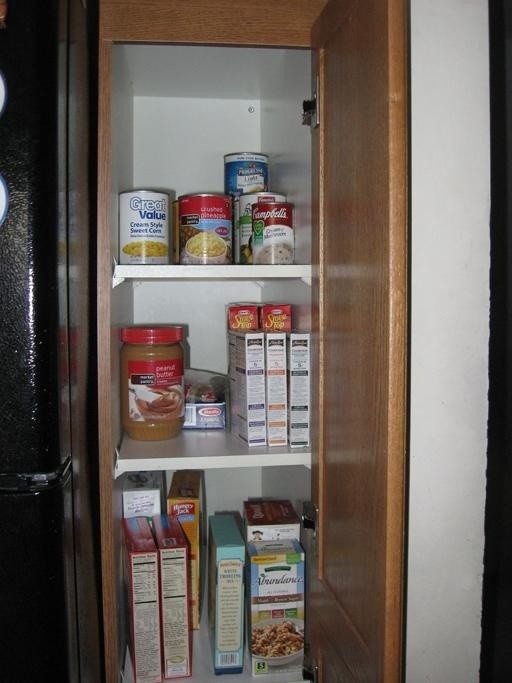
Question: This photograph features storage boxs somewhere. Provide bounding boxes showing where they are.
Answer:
[120,472,305,683]
[231,331,309,449]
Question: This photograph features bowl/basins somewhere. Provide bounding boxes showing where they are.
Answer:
[251,618,304,666]
[185,237,227,264]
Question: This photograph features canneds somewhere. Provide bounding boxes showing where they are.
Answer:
[117,153,294,264]
[117,326,186,441]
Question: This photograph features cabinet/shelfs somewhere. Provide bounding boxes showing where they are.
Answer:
[95,0,407,682]
